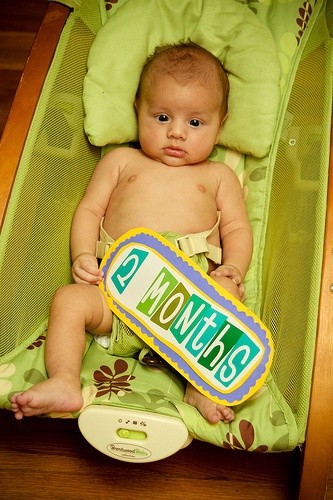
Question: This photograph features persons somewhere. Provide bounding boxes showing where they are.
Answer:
[11,39,253,424]
